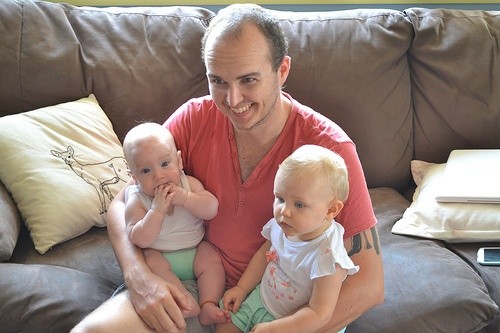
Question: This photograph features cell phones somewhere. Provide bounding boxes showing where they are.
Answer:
[477,246,500,266]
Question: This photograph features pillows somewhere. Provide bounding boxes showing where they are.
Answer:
[0,93,132,255]
[390,159,500,243]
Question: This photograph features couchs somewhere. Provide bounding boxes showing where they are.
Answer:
[0,0,500,333]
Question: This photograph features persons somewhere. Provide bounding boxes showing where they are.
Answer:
[123,122,232,325]
[70,3,384,333]
[214,144,360,333]
